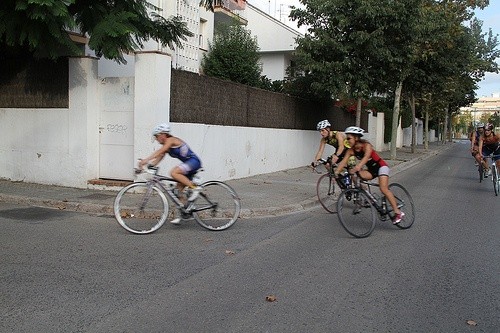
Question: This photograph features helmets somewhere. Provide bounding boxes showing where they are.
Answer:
[477,122,484,127]
[317,119,331,130]
[344,126,364,136]
[483,123,493,130]
[153,124,170,135]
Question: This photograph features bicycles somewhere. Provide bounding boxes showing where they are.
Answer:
[473,148,500,196]
[312,155,371,213]
[112,158,241,235]
[335,171,415,238]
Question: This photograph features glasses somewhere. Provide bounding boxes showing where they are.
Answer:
[347,138,354,140]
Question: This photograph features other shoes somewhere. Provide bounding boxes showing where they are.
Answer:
[188,186,203,202]
[171,218,182,225]
[484,170,489,177]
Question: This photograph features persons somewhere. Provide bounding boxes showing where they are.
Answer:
[138,122,202,224]
[312,119,356,178]
[334,126,405,224]
[471,120,500,178]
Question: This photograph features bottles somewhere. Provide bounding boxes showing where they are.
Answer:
[345,171,350,188]
[170,184,180,199]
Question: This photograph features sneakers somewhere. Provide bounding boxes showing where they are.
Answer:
[392,212,405,224]
[363,200,374,206]
